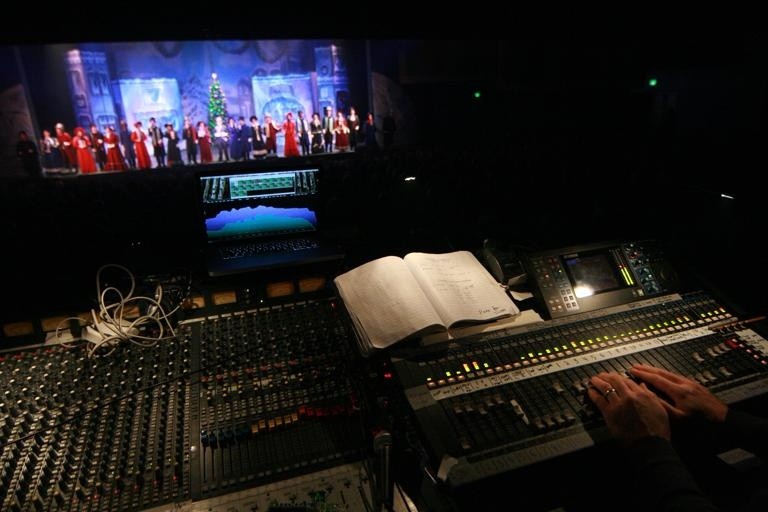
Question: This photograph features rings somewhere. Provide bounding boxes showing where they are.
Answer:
[605,389,615,397]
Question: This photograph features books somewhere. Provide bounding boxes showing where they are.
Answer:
[333,249,520,360]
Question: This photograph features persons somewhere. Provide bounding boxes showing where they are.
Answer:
[16,106,362,176]
[588,364,767,512]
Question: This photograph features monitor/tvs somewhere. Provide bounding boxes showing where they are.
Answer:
[16,23,383,192]
